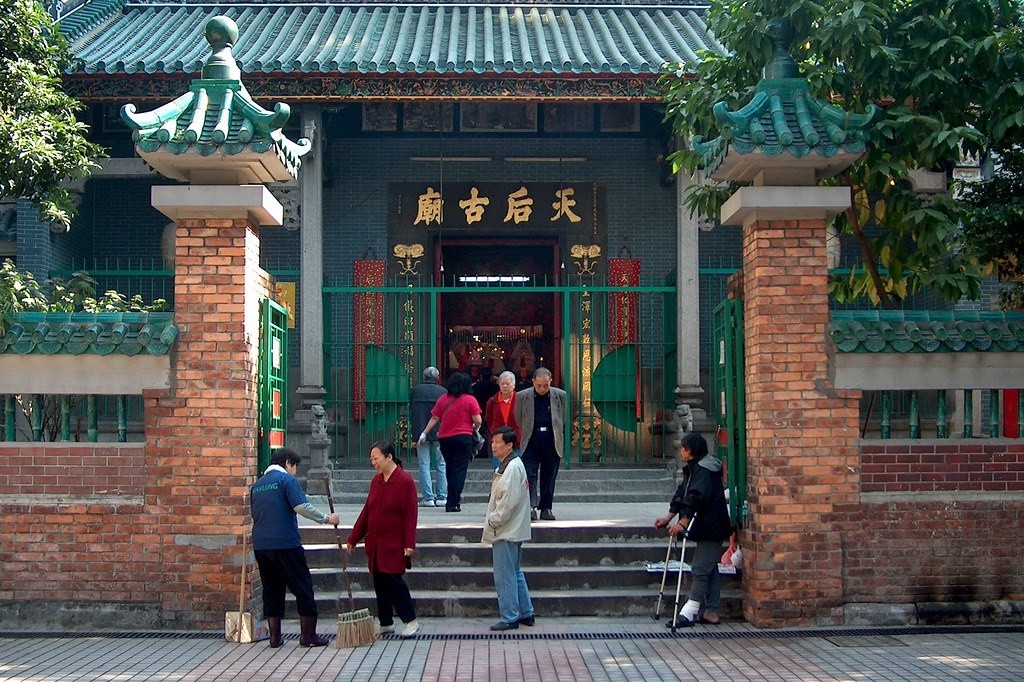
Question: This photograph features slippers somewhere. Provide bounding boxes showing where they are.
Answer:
[695,612,721,624]
[666,615,695,627]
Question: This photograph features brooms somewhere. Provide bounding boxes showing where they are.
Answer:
[323,471,377,649]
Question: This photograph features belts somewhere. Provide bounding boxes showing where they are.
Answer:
[533,427,552,431]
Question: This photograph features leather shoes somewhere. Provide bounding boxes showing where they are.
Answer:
[531,509,537,520]
[519,615,536,626]
[446,505,461,512]
[490,620,519,630]
[540,509,555,520]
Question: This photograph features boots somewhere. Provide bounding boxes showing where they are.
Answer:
[268,617,284,648]
[300,615,329,646]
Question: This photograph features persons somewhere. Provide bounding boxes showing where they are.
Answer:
[479,426,535,631]
[410,367,447,507]
[251,447,340,648]
[515,367,567,521]
[417,371,482,512]
[484,370,522,473]
[346,440,420,637]
[654,432,731,628]
[469,365,534,414]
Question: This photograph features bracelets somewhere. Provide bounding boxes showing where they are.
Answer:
[679,520,688,528]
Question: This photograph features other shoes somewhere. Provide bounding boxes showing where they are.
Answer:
[377,624,395,634]
[402,620,420,635]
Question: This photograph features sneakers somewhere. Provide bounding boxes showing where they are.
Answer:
[418,498,435,507]
[437,499,447,506]
